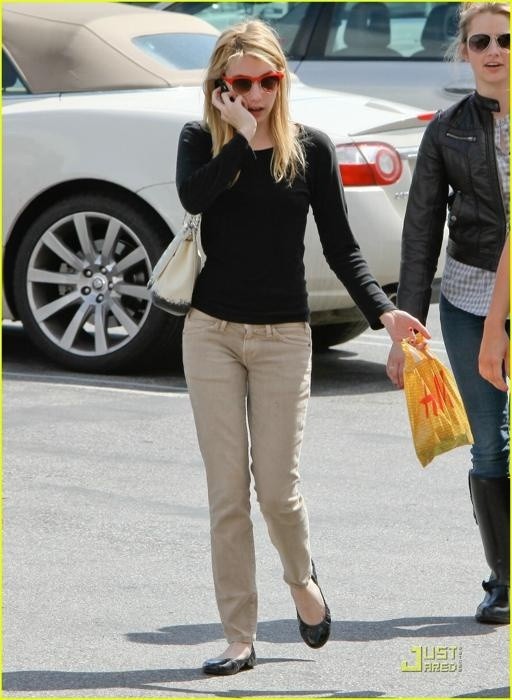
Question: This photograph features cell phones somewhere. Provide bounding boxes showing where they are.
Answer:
[214,79,235,102]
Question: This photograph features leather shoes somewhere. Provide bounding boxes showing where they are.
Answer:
[203,642,258,674]
[298,559,330,648]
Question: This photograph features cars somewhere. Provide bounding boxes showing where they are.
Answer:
[135,0,478,116]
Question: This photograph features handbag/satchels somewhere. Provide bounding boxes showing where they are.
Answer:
[146,212,207,317]
[402,332,473,467]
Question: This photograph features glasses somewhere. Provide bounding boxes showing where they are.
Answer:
[221,70,284,95]
[467,33,509,54]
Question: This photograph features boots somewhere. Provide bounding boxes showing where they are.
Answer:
[467,470,509,625]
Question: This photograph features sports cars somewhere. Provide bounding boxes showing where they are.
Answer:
[2,2,448,375]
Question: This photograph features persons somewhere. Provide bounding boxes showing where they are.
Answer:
[478,226,510,390]
[385,2,509,625]
[174,22,432,675]
